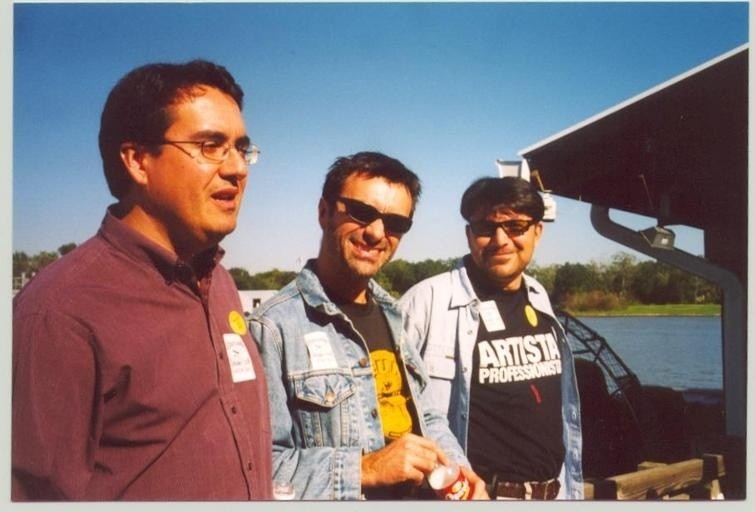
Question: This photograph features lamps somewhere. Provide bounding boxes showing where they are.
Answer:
[638,216,676,252]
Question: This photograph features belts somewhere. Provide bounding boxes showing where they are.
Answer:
[485,478,563,502]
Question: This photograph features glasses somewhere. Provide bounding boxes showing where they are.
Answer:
[466,216,538,236]
[332,195,415,235]
[140,134,263,165]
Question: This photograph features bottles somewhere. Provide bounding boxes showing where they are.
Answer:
[426,458,474,502]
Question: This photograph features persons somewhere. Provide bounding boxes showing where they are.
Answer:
[240,153,492,501]
[388,175,588,500]
[13,56,278,501]
